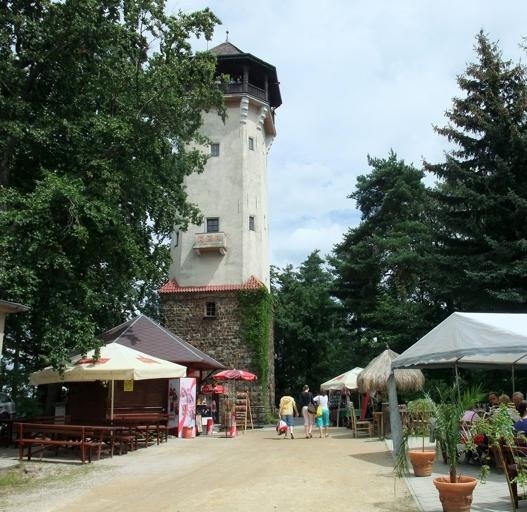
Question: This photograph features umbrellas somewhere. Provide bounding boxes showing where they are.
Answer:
[25,343,187,439]
[212,368,258,435]
[199,383,229,435]
[320,366,364,420]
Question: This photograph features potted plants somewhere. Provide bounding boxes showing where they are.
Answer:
[394,380,512,511]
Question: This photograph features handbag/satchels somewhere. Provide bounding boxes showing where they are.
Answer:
[308,402,317,415]
[276,420,289,435]
[314,404,322,418]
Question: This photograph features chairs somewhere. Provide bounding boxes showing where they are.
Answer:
[349,404,371,438]
[494,435,526,511]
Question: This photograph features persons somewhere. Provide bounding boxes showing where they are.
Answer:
[278,388,301,440]
[459,389,526,469]
[210,400,217,422]
[298,384,316,439]
[312,389,332,438]
